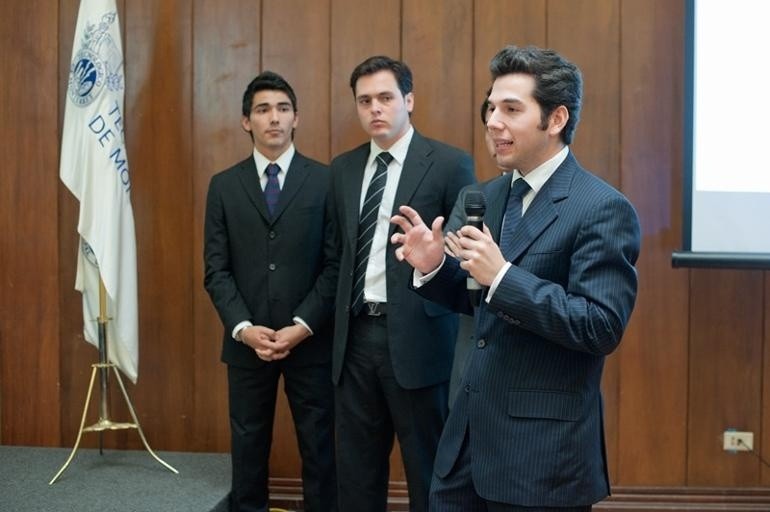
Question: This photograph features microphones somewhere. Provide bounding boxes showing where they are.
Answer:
[464,190,487,290]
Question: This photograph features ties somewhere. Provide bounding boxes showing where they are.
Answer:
[348,152,394,317]
[499,179,531,251]
[264,164,281,214]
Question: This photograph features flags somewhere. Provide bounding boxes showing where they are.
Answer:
[58,0,138,385]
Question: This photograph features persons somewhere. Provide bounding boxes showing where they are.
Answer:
[390,47,642,512]
[204,70,338,512]
[480,86,518,175]
[329,57,473,512]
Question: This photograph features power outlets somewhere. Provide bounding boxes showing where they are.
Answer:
[722,431,753,452]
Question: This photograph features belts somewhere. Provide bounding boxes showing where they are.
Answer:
[353,299,393,319]
